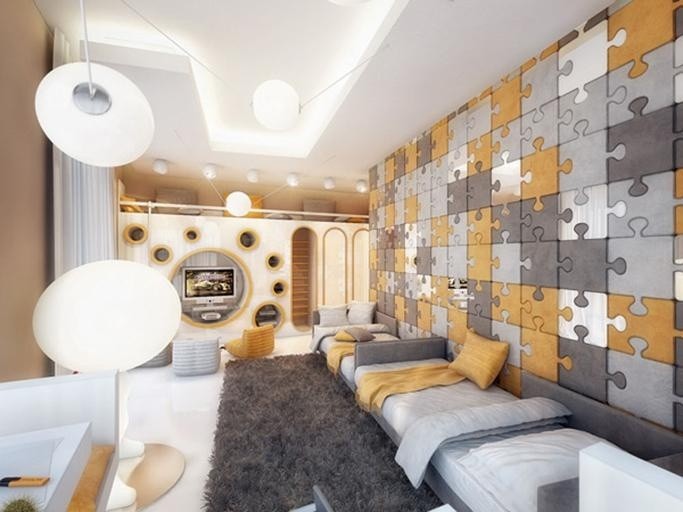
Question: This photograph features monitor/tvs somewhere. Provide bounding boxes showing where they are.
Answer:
[182,267,236,311]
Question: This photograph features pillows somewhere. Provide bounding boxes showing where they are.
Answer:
[348,300,377,325]
[335,330,357,342]
[448,330,508,389]
[319,305,349,328]
[345,326,376,342]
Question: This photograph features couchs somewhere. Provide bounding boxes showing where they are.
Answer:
[353,338,683,512]
[311,306,445,394]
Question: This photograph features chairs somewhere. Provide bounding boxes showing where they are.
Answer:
[139,342,171,367]
[219,325,275,360]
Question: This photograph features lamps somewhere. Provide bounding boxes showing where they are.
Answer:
[194,161,337,218]
[34,0,155,167]
[32,259,185,511]
[152,158,167,175]
[356,180,368,193]
[120,0,391,130]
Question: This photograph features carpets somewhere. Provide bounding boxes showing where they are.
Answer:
[202,353,445,512]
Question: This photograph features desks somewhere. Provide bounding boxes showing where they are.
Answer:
[0,376,117,512]
[172,334,220,377]
